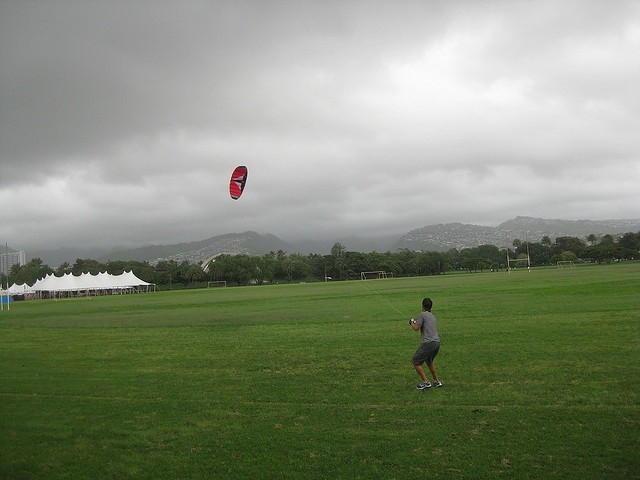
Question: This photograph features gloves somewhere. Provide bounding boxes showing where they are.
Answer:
[409,318,414,324]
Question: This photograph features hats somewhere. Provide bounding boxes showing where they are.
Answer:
[422,297,433,310]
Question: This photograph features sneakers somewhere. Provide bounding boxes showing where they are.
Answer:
[434,381,443,388]
[415,381,432,390]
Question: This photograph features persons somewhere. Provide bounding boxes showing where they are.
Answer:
[409,298,443,390]
